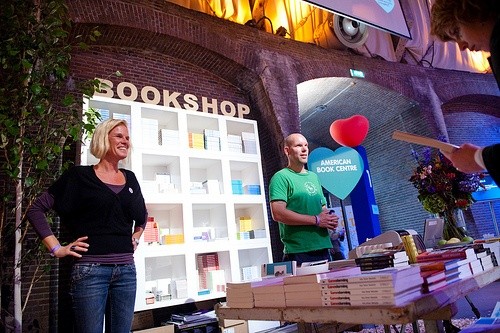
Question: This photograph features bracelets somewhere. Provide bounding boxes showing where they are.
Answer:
[315,216,320,225]
[50,244,61,252]
[134,239,139,245]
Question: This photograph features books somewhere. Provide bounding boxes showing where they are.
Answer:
[142,117,267,302]
[225,238,500,307]
[391,130,460,153]
[92,108,131,127]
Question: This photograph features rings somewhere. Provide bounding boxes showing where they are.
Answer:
[71,246,76,250]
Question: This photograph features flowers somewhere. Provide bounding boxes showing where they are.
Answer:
[402,133,489,218]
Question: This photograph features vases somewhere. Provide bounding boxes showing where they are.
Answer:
[436,209,464,242]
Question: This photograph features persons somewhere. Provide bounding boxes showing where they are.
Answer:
[269,133,339,266]
[430,0,500,188]
[25,118,149,333]
[328,210,346,261]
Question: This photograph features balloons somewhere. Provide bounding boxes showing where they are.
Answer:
[330,115,369,147]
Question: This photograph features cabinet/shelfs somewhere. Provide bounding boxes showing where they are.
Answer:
[80,93,273,312]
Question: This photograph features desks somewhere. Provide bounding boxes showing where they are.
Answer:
[214,264,500,333]
